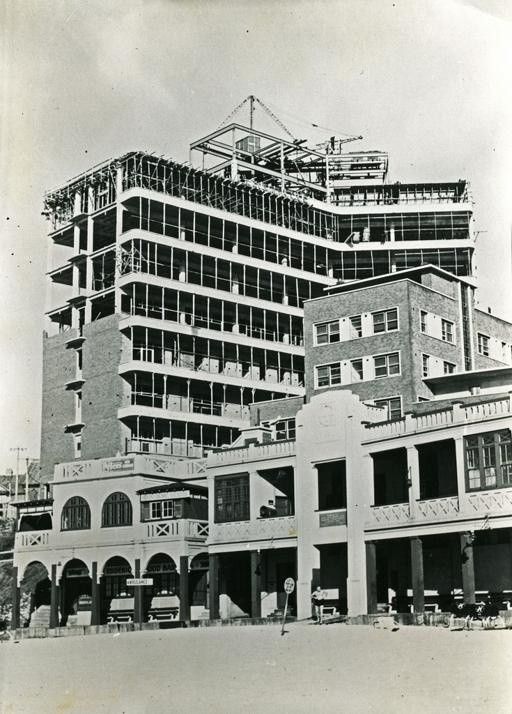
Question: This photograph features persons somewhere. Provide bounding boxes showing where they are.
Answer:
[260,499,277,519]
[310,585,328,626]
